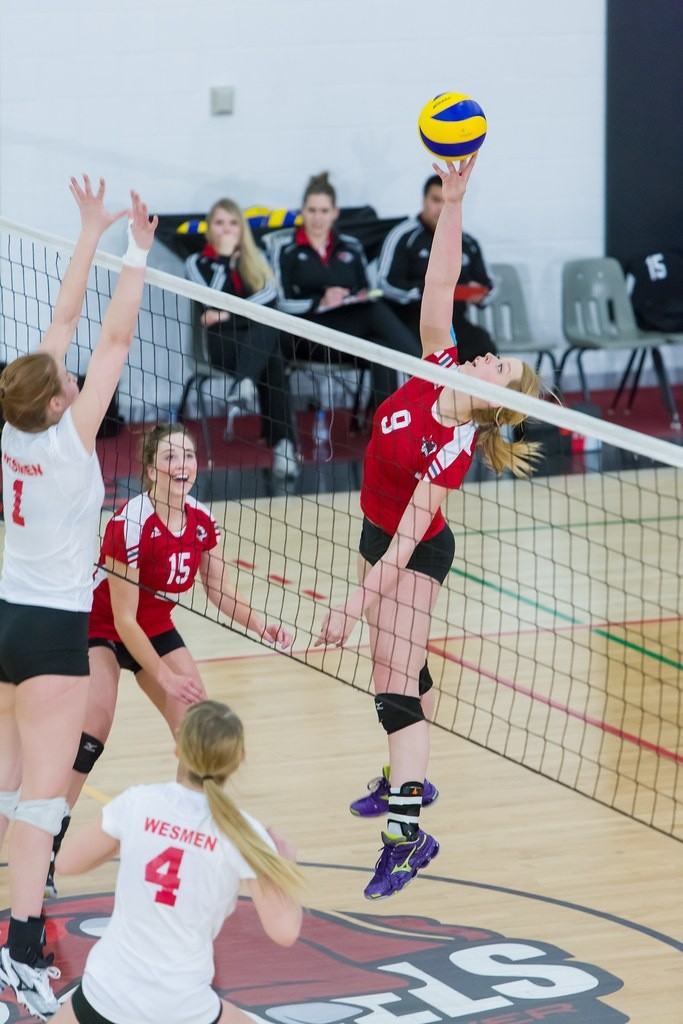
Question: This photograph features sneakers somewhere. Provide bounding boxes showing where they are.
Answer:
[273,439,299,481]
[364,827,441,901]
[350,765,439,818]
[0,946,61,1022]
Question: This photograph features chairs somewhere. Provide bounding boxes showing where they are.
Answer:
[553,256,683,432]
[262,228,371,441]
[464,262,560,405]
[174,296,306,472]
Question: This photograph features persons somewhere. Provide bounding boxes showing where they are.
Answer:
[0,176,158,1023]
[315,150,544,902]
[184,199,302,480]
[273,173,422,401]
[45,426,294,899]
[379,176,497,363]
[43,702,304,1023]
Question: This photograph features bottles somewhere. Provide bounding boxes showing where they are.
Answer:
[311,411,329,463]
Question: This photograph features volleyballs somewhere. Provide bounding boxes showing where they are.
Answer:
[416,92,486,163]
